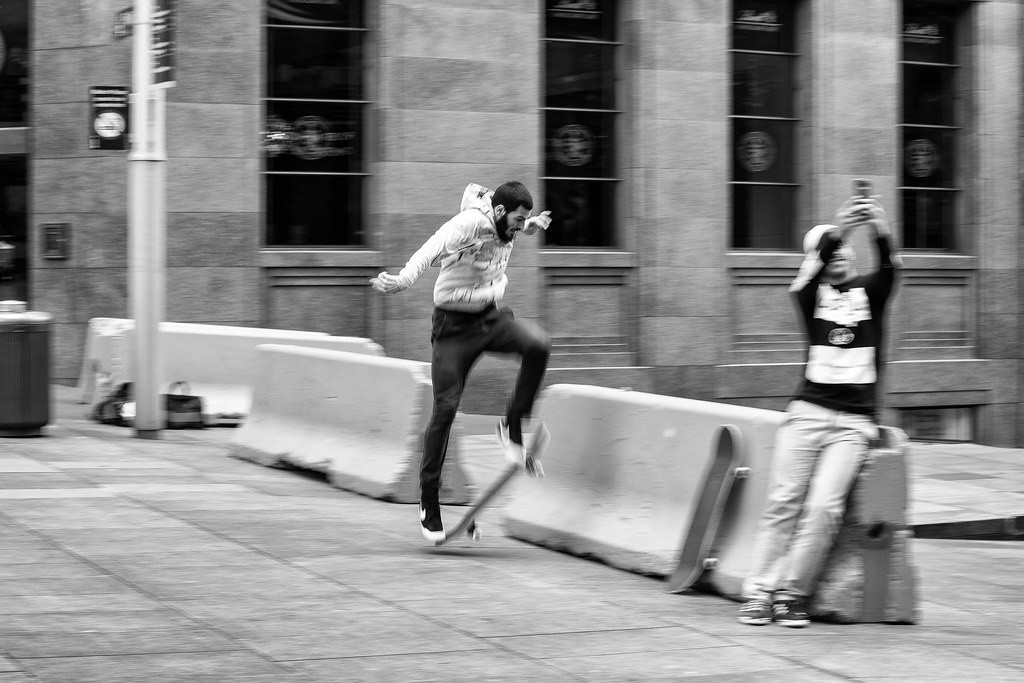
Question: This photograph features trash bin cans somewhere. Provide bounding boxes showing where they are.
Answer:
[0,299,54,438]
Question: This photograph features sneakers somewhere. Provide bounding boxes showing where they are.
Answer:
[419,492,445,540]
[497,418,524,465]
[737,599,811,626]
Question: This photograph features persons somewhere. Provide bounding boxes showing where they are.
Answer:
[735,192,907,626]
[365,177,554,544]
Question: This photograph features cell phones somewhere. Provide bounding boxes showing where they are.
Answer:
[853,178,872,222]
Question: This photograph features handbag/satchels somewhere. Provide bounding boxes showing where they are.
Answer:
[162,381,203,430]
[95,382,136,427]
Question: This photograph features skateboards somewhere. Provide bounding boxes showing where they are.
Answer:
[433,419,552,549]
[659,423,753,594]
